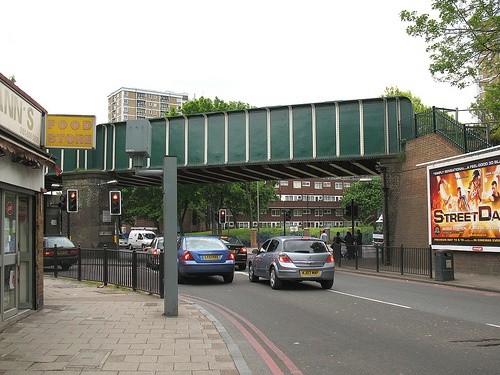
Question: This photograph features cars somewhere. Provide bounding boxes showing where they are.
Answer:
[205,235,247,270]
[118,234,128,248]
[144,237,164,270]
[42,237,79,270]
[160,236,234,282]
[248,236,336,290]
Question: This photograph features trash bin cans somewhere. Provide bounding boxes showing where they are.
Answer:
[434,249,454,282]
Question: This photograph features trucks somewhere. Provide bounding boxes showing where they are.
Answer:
[372,220,384,247]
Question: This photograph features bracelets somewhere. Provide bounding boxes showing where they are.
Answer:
[468,188,471,193]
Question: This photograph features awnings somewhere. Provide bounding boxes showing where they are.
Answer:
[0,134,56,169]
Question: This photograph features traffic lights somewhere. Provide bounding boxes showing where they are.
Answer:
[58,194,66,211]
[109,191,121,216]
[346,204,352,217]
[285,210,292,222]
[219,209,226,225]
[66,189,78,213]
[352,205,359,218]
[215,212,219,222]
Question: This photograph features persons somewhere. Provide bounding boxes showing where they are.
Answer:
[433,179,456,212]
[457,186,471,212]
[482,175,500,210]
[321,230,327,244]
[344,232,356,259]
[354,229,363,258]
[333,232,346,244]
[468,168,483,207]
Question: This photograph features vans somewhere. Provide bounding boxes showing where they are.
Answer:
[128,230,156,250]
